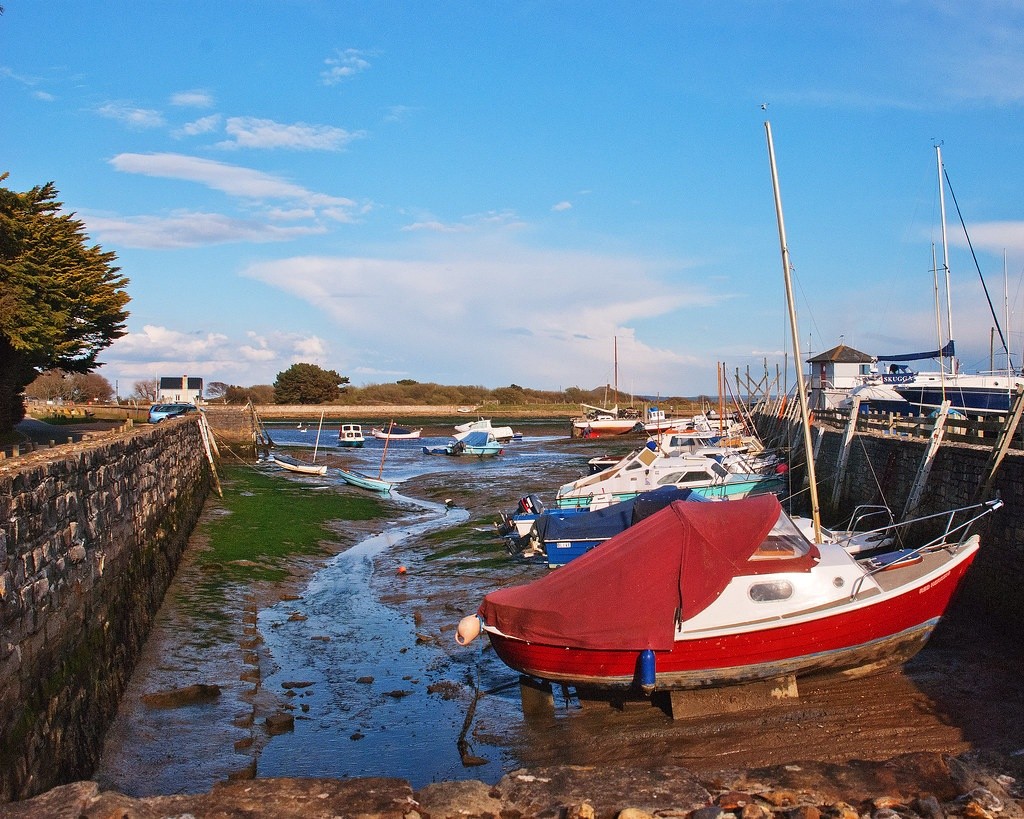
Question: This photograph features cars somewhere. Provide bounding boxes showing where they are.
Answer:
[26,396,47,405]
[164,405,205,421]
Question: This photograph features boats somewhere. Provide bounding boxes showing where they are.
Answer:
[454,419,523,444]
[372,422,421,440]
[422,430,504,457]
[337,423,365,448]
[456,119,1004,694]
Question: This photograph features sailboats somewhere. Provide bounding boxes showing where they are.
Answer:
[841,142,1024,414]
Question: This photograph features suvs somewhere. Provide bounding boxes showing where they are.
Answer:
[148,404,202,422]
